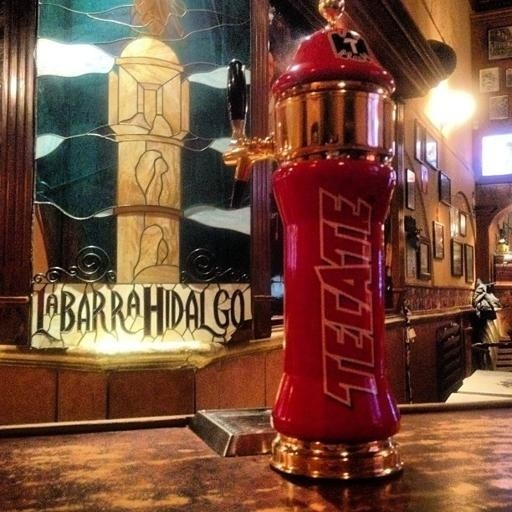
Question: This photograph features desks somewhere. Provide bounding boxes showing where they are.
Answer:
[442,370,512,404]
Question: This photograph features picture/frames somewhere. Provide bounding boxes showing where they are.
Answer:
[478,26,512,121]
[405,118,476,284]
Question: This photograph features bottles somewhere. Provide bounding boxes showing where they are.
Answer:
[272,0,404,482]
[228,59,248,142]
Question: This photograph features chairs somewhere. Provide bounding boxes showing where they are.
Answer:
[470,341,512,371]
